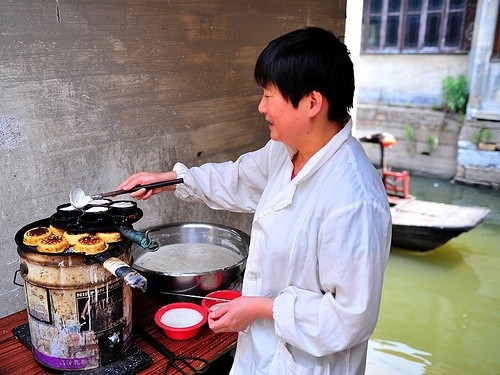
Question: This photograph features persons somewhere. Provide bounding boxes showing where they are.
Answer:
[110,26,393,375]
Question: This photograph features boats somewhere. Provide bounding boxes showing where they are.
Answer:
[381,193,491,255]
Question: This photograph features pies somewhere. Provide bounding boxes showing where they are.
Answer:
[22,224,121,255]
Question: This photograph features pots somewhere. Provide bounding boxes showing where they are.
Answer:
[49,201,161,251]
[15,216,148,293]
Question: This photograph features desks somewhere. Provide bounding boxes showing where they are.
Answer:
[0,308,238,375]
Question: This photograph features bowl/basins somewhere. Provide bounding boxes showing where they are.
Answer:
[84,197,112,209]
[200,290,243,314]
[81,205,110,219]
[56,202,83,217]
[154,302,208,341]
[131,221,251,296]
[110,200,137,217]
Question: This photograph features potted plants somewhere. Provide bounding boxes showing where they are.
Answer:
[476,129,500,151]
